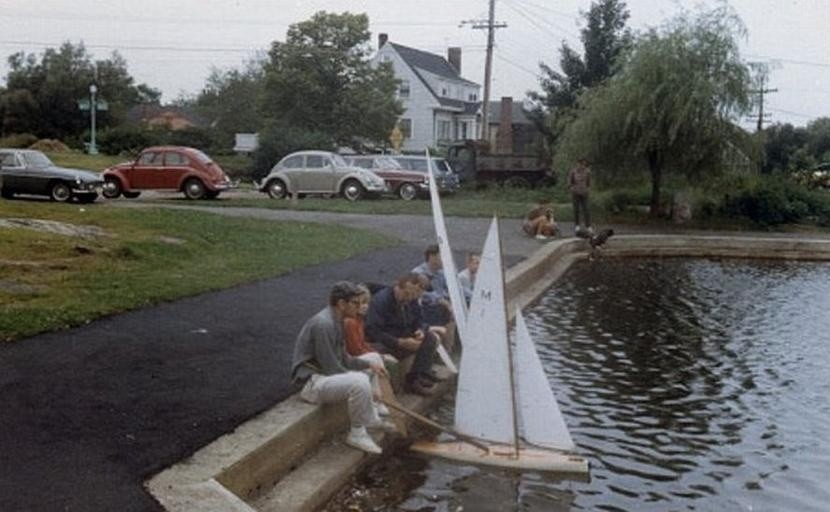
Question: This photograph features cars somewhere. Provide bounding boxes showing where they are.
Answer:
[251,148,459,204]
[0,145,105,204]
[99,145,240,200]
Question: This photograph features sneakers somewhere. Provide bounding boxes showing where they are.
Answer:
[344,431,383,454]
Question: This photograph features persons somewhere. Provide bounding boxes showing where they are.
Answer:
[523,197,550,240]
[568,157,592,234]
[588,228,614,262]
